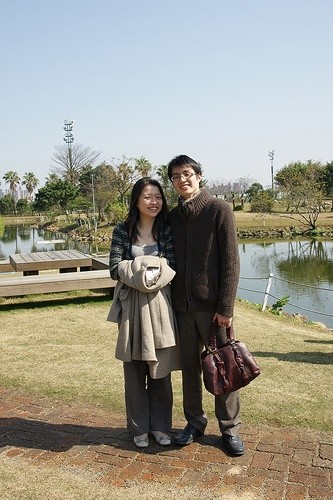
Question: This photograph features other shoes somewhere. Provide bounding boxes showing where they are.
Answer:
[134,433,149,449]
[150,431,171,444]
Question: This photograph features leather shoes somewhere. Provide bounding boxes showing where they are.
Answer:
[222,433,245,454]
[174,423,204,445]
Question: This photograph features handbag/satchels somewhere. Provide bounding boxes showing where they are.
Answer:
[201,318,261,396]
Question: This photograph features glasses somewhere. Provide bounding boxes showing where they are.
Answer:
[171,172,201,181]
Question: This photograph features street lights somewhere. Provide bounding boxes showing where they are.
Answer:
[62,118,77,180]
[268,148,275,192]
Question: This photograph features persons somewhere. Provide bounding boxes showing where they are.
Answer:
[108,176,175,448]
[164,153,246,455]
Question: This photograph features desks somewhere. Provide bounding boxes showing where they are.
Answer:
[9,250,93,276]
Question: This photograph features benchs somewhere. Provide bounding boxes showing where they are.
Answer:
[0,258,118,297]
